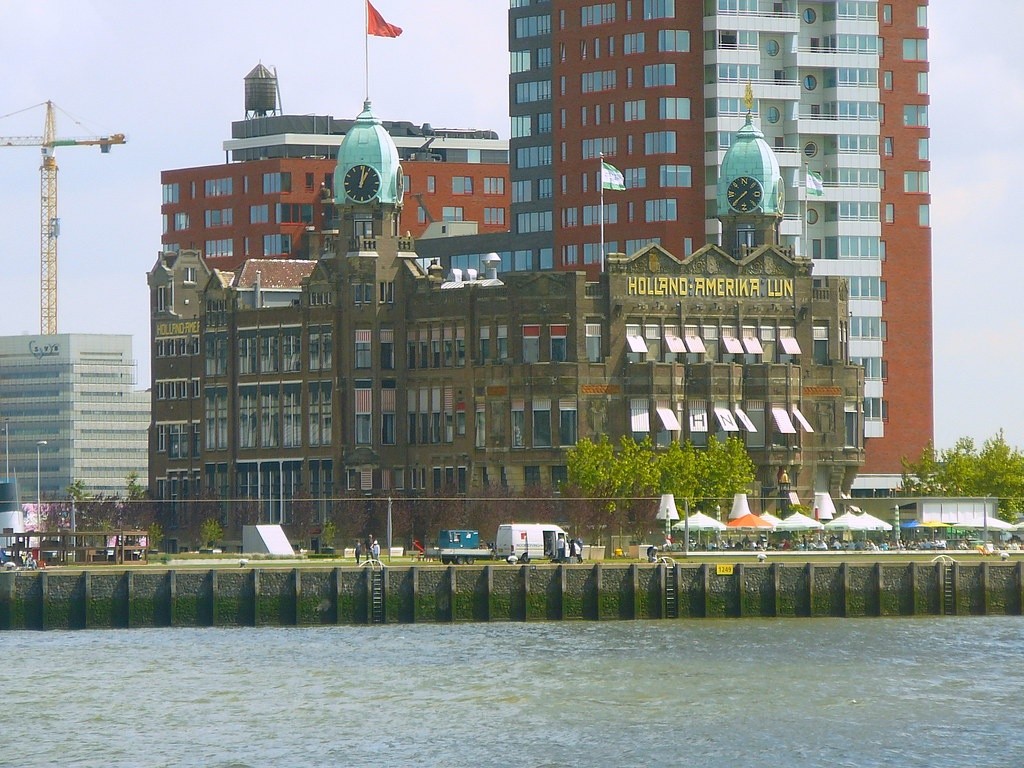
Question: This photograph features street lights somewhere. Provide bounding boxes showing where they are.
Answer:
[36,441,47,559]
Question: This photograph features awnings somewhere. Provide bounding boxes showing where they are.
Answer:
[657,408,815,433]
[626,335,802,354]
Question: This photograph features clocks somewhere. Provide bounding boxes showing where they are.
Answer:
[342,164,381,204]
[726,176,764,214]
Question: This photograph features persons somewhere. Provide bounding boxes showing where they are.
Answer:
[558,536,564,563]
[1006,538,1017,550]
[577,538,583,563]
[25,553,33,562]
[649,546,658,563]
[373,540,380,560]
[364,534,374,563]
[900,538,932,550]
[355,540,361,563]
[570,540,576,557]
[679,535,888,551]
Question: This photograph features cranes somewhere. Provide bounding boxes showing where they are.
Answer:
[0,99,127,335]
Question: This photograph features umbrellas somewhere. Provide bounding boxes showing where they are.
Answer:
[671,510,893,543]
[900,520,922,541]
[952,517,1017,532]
[916,520,950,541]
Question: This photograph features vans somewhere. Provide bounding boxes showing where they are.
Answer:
[496,524,581,564]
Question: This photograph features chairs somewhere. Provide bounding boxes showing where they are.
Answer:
[36,560,46,569]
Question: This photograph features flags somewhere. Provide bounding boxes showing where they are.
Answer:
[602,162,626,191]
[807,170,823,196]
[368,0,403,38]
